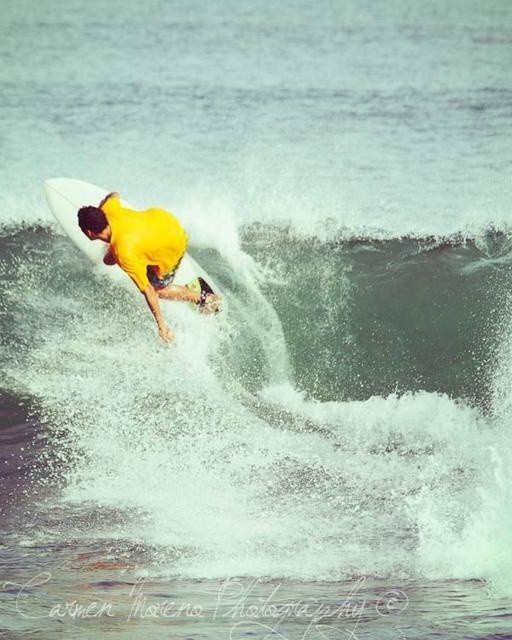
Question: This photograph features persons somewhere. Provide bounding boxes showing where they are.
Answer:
[76,190,221,343]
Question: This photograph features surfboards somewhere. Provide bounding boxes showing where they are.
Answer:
[42,178,228,328]
